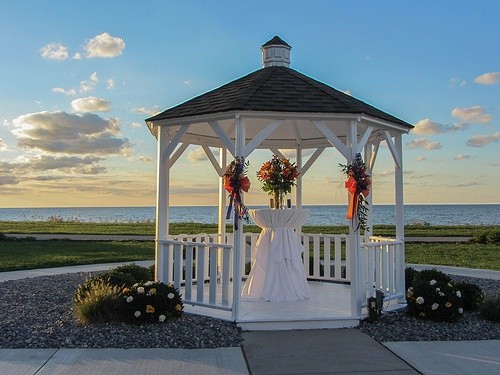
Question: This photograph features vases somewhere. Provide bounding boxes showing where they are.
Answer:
[273,190,286,210]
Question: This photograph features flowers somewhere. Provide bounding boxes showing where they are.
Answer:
[122,280,183,323]
[222,156,251,193]
[339,152,371,197]
[256,155,300,191]
[406,271,464,320]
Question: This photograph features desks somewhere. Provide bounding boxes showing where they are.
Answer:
[240,209,308,301]
